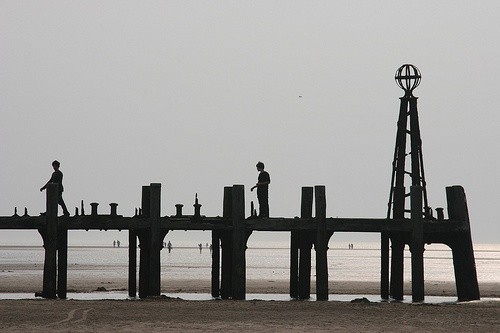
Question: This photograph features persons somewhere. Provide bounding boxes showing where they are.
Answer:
[349,244,353,249]
[40,160,70,216]
[251,162,270,217]
[198,243,202,253]
[167,241,172,253]
[113,240,120,248]
